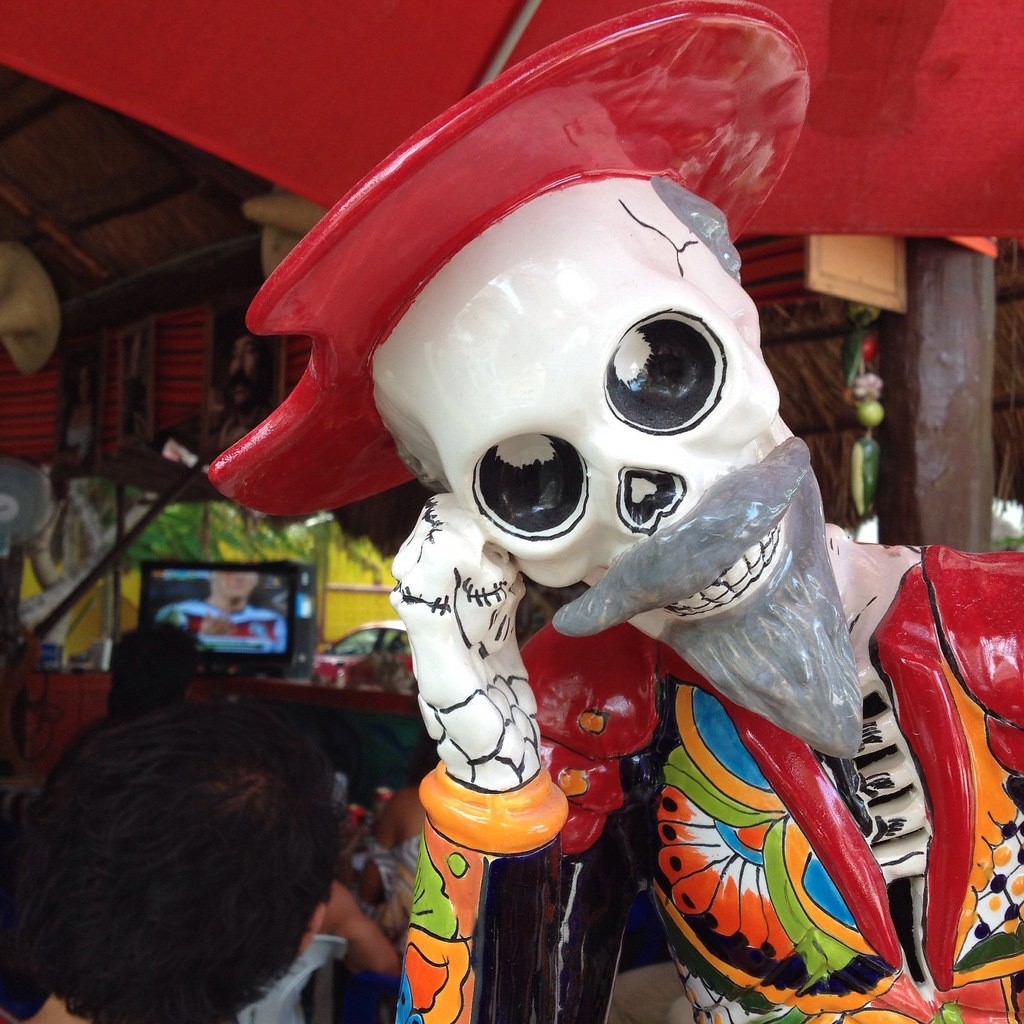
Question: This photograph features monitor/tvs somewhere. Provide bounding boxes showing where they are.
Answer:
[138,557,315,676]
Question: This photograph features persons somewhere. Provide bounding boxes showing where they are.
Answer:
[2,224,451,1024]
